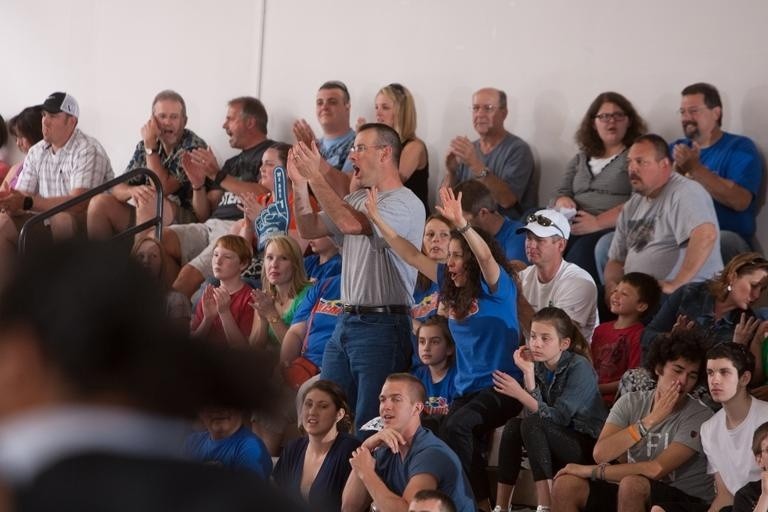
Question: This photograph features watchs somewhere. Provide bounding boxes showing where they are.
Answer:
[145,149,157,155]
[474,166,491,180]
[458,221,472,233]
[267,314,281,324]
[215,170,228,185]
[23,196,33,211]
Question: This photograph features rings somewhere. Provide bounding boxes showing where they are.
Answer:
[201,159,205,163]
[294,155,300,158]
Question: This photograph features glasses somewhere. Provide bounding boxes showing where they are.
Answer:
[527,214,565,240]
[468,105,503,113]
[593,112,628,121]
[350,143,389,153]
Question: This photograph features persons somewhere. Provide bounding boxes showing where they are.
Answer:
[363,185,536,483]
[413,315,498,503]
[551,334,716,511]
[1,117,12,185]
[130,235,192,331]
[595,83,766,288]
[491,308,619,512]
[700,342,768,511]
[294,80,357,173]
[413,214,458,353]
[87,89,217,241]
[286,124,427,443]
[612,252,768,413]
[342,374,479,512]
[269,381,360,511]
[149,97,293,304]
[231,142,323,247]
[440,90,537,227]
[358,83,429,215]
[248,236,314,353]
[733,421,767,511]
[547,92,650,323]
[301,235,342,283]
[609,134,725,307]
[188,234,256,351]
[511,209,600,362]
[184,408,272,480]
[590,272,663,411]
[253,276,342,454]
[0,105,45,194]
[1,92,115,239]
[409,491,459,512]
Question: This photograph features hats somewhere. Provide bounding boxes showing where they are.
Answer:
[32,92,79,119]
[516,209,571,240]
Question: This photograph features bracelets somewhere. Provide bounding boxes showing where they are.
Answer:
[591,462,610,481]
[636,420,648,437]
[193,184,205,191]
[629,424,640,442]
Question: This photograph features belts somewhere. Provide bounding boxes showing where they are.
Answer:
[343,304,409,314]
